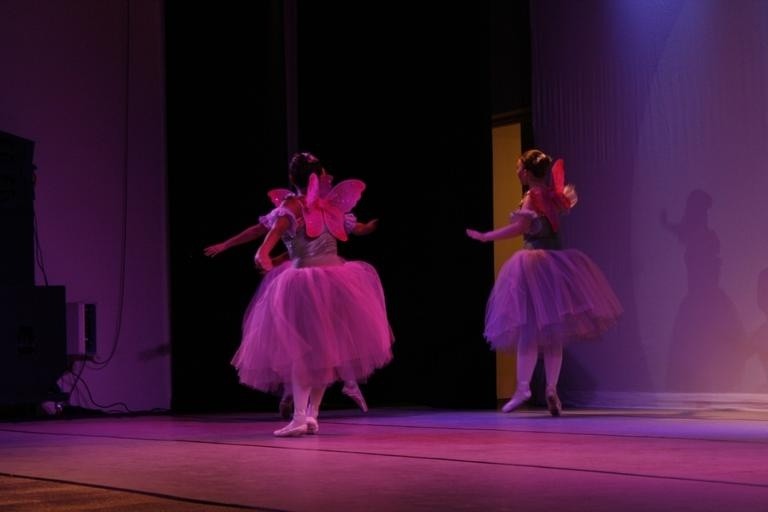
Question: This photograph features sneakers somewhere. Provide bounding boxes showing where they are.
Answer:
[545,385,562,416]
[273,424,308,437]
[502,390,532,413]
[342,386,368,413]
[280,399,291,418]
[306,416,319,434]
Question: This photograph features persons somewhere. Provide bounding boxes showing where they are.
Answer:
[464,149,627,417]
[227,149,398,436]
[199,168,371,415]
